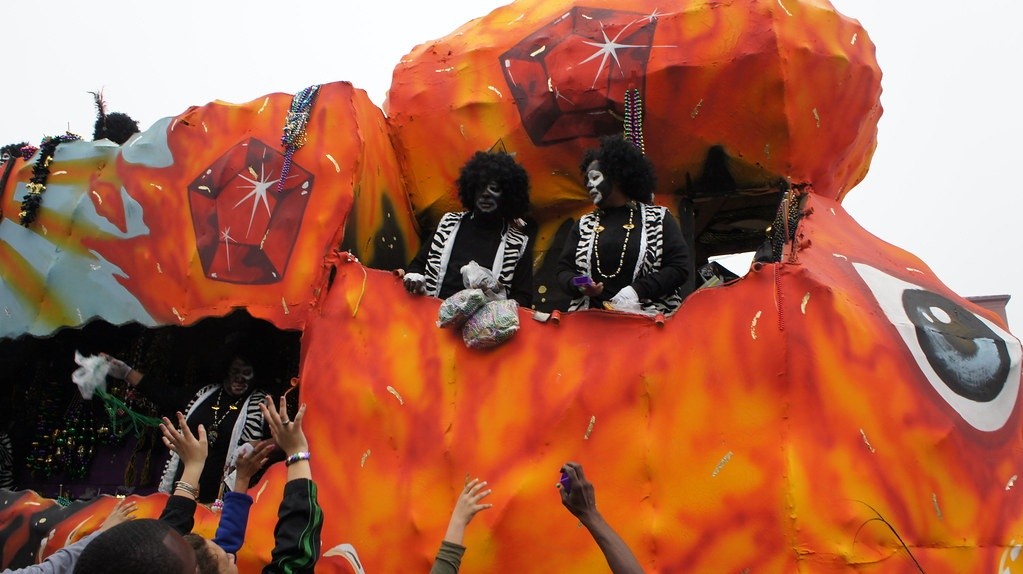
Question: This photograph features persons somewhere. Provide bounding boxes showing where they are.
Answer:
[101,347,269,508]
[73,518,200,574]
[0,426,21,492]
[430,478,492,574]
[403,150,534,308]
[556,139,690,315]
[559,461,644,574]
[159,411,208,536]
[212,440,276,564]
[2,499,138,574]
[183,395,324,574]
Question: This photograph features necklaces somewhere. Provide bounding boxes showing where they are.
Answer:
[211,388,242,428]
[594,208,634,278]
[277,86,321,192]
[622,88,645,156]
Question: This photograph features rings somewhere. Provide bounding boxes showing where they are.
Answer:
[283,420,289,425]
[170,438,175,444]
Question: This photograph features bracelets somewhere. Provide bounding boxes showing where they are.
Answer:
[285,452,310,467]
[175,481,199,498]
